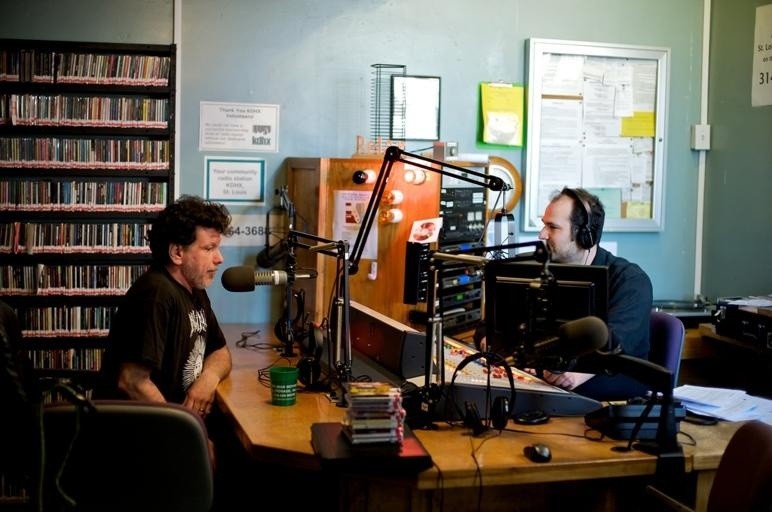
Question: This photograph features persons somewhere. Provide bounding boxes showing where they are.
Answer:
[93,195,232,472]
[473,185,653,404]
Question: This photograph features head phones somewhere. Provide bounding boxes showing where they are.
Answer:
[563,185,598,249]
[449,351,517,432]
[274,291,303,340]
[295,321,325,384]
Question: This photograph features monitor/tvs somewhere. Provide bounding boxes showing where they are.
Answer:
[484,261,608,393]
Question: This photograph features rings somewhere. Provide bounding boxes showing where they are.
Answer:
[200,408,204,412]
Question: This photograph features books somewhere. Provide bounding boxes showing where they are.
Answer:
[0,51,171,375]
[340,379,406,446]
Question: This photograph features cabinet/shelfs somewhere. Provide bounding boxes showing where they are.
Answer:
[283,153,441,343]
[1,39,177,410]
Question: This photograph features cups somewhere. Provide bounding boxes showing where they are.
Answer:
[268,366,300,407]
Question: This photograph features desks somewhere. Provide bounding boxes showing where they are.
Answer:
[682,305,768,363]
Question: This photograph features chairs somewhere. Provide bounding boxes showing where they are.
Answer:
[1,304,97,460]
[647,420,771,512]
[39,404,214,511]
[650,309,685,399]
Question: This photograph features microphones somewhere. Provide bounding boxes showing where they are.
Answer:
[254,235,297,264]
[527,315,610,359]
[219,265,312,292]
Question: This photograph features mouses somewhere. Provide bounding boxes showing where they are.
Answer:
[524,441,555,462]
